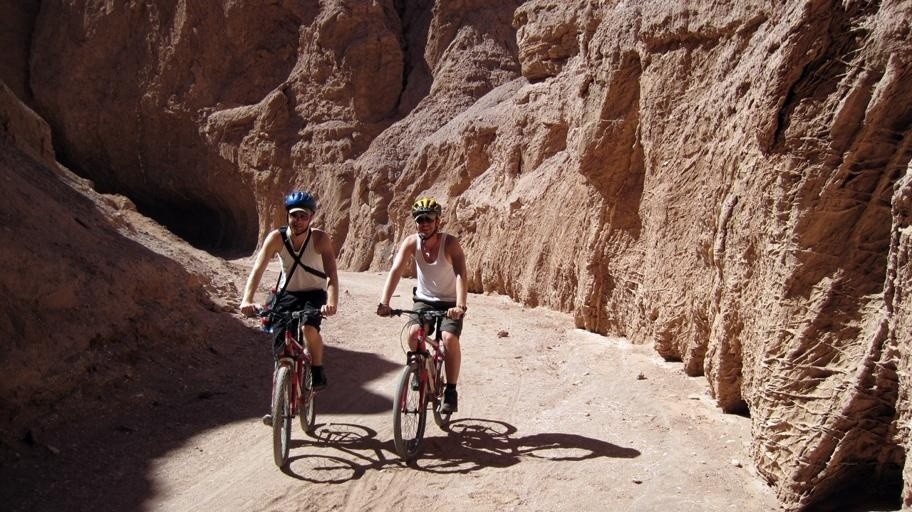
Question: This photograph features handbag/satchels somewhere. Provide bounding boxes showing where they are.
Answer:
[259,300,276,331]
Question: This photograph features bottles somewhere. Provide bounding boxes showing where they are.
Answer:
[427,349,435,378]
[262,289,277,329]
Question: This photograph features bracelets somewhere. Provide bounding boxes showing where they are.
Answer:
[456,304,466,311]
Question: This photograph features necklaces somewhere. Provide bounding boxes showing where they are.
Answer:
[421,242,437,257]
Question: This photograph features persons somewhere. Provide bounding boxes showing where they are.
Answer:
[377,199,468,413]
[240,191,339,427]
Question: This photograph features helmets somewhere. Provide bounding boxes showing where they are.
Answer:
[411,198,441,222]
[284,192,316,215]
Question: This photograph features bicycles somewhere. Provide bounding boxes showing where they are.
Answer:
[377,305,463,456]
[246,305,337,467]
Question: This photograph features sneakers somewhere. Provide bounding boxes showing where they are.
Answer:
[310,365,327,393]
[411,371,420,392]
[439,387,458,414]
[263,413,272,426]
